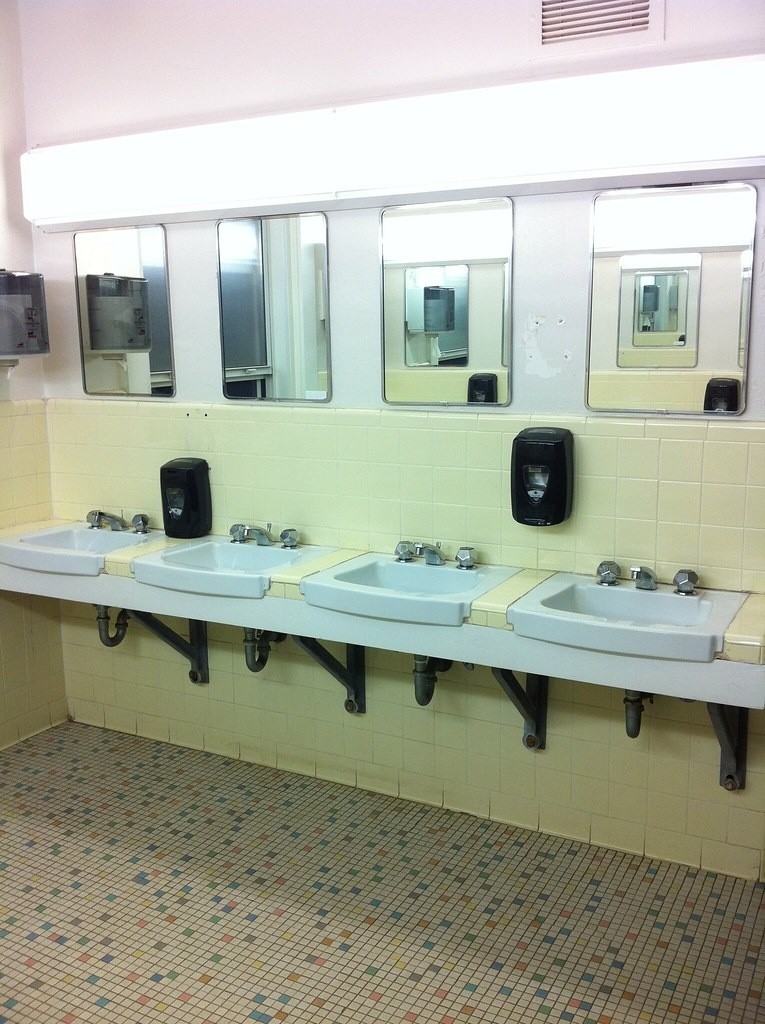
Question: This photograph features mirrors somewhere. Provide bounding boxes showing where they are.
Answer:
[640,275,679,331]
[584,183,759,417]
[72,224,175,399]
[403,263,470,369]
[618,252,702,367]
[378,197,513,408]
[633,269,689,346]
[216,212,333,403]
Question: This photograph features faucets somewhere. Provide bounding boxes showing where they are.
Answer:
[230,524,274,547]
[88,510,129,531]
[629,567,656,589]
[413,543,445,566]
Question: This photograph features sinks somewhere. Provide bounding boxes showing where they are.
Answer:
[300,560,486,626]
[0,528,147,578]
[506,581,720,661]
[135,541,299,599]
